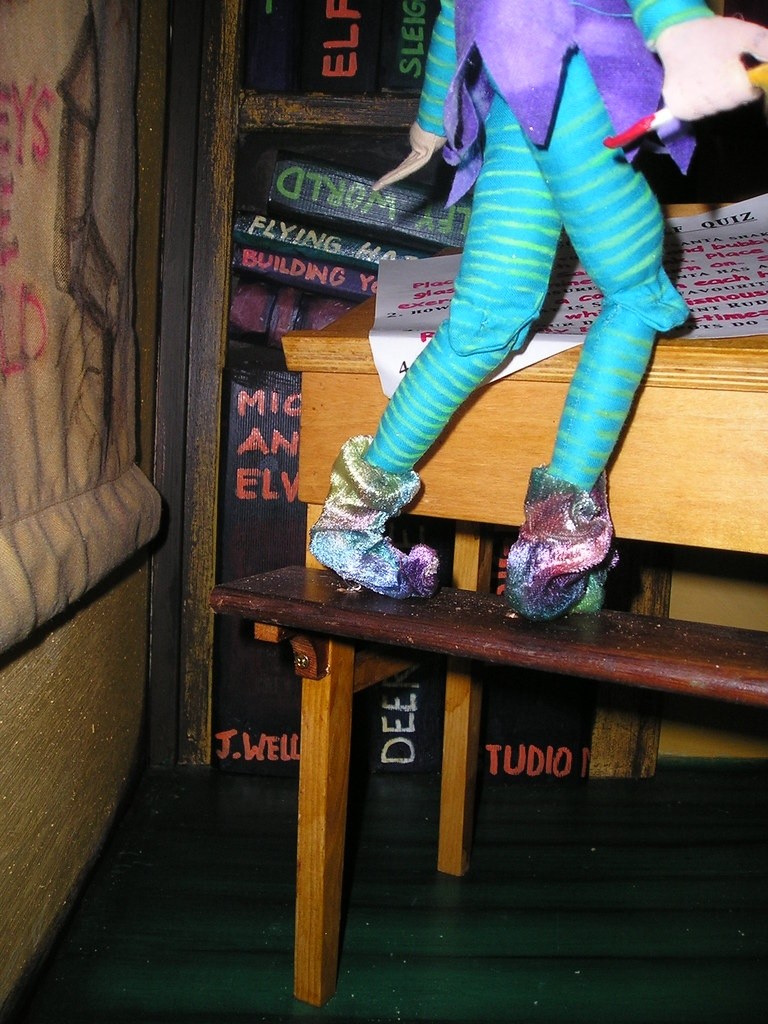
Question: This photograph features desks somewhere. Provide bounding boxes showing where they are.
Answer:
[276,200,768,1012]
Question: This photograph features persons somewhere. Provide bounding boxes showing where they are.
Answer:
[307,0,768,619]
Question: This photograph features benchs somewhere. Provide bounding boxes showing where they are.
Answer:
[208,562,768,1007]
